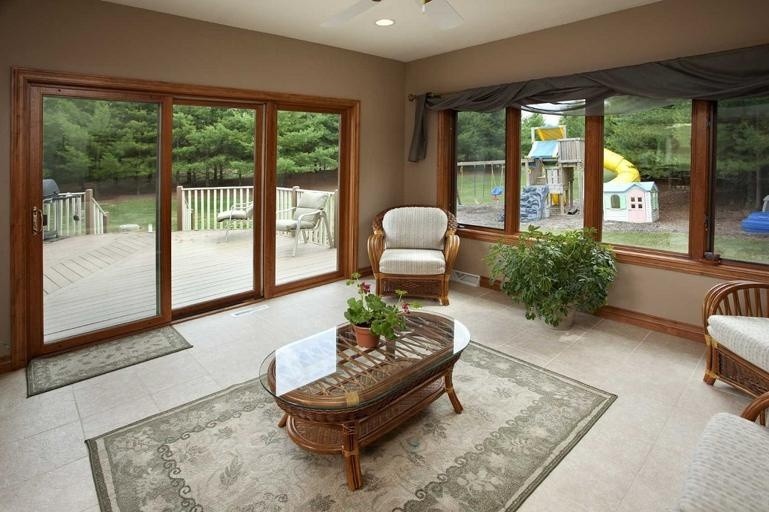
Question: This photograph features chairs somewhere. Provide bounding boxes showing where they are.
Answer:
[217,203,253,240]
[701,273,768,402]
[368,202,461,307]
[674,392,767,511]
[274,191,334,257]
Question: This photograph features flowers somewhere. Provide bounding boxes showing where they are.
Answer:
[344,266,413,339]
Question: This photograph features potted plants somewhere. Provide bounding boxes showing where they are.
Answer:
[478,221,618,330]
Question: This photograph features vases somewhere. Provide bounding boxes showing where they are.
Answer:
[349,322,379,348]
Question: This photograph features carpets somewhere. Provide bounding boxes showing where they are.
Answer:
[82,340,618,512]
[25,324,194,399]
